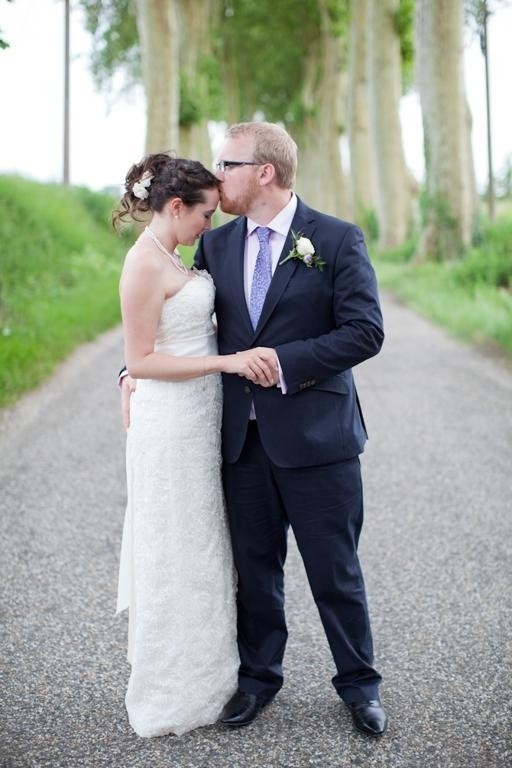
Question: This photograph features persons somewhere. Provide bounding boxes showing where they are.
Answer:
[119,123,387,737]
[113,153,279,737]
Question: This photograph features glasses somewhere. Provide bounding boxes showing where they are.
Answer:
[213,158,255,173]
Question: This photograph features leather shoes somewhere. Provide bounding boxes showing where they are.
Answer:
[218,686,276,728]
[345,695,389,737]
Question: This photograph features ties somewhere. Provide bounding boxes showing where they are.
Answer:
[249,225,273,333]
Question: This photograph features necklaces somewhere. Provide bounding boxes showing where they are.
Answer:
[144,225,188,275]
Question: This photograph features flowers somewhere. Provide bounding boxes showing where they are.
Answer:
[130,169,156,202]
[278,226,325,271]
